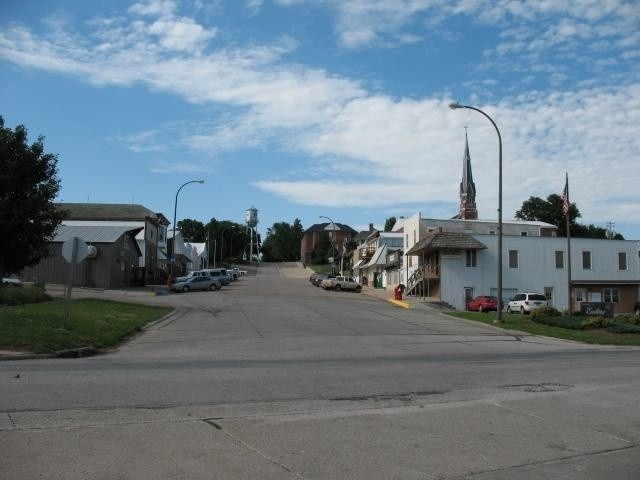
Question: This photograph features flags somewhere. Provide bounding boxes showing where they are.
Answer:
[560,173,571,217]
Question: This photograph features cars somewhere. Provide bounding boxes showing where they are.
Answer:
[309,272,362,293]
[466,296,504,313]
[171,268,248,292]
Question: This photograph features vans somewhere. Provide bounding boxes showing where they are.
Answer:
[507,293,548,315]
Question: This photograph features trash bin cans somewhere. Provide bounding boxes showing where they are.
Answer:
[394,287,402,300]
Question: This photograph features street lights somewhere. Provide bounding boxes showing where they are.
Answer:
[319,216,335,274]
[449,102,503,322]
[173,179,204,255]
[221,226,236,268]
